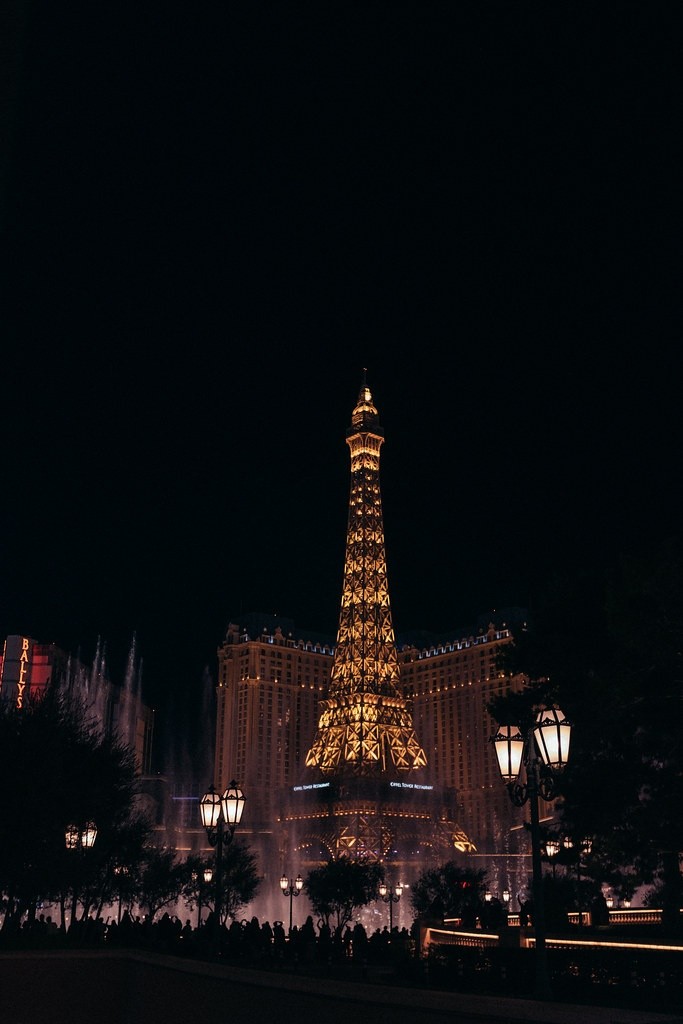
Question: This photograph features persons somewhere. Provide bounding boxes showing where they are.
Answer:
[19,908,411,957]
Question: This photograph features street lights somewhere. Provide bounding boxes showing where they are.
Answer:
[279,872,306,934]
[199,777,247,963]
[64,817,98,926]
[494,708,572,1004]
[378,880,403,934]
